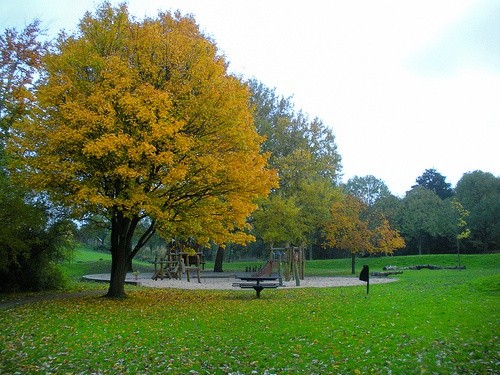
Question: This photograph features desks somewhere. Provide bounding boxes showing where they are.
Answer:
[170,251,204,283]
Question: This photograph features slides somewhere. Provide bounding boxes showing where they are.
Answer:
[256,261,275,276]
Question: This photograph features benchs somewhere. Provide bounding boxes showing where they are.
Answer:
[149,260,179,281]
[239,276,282,284]
[231,282,278,299]
